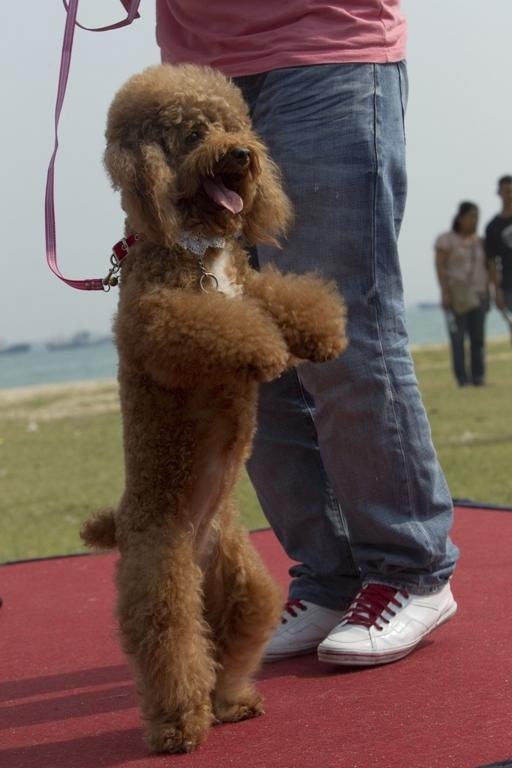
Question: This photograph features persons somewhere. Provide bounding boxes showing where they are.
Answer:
[432,199,494,388]
[146,0,461,666]
[481,175,511,325]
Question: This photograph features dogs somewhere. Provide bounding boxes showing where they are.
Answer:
[79,61,351,754]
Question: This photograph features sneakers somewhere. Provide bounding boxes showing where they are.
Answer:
[243,584,364,668]
[315,577,460,667]
